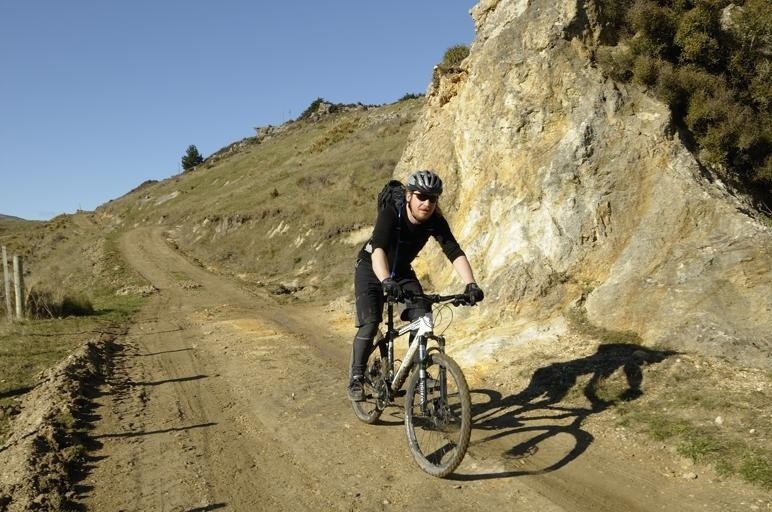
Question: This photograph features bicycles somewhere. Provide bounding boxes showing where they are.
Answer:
[345,282,477,477]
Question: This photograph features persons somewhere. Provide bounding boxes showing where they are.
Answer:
[346,170,484,402]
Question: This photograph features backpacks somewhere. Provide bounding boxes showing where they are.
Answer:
[378,180,406,219]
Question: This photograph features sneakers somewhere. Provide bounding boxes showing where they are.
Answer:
[346,374,366,401]
[418,370,441,388]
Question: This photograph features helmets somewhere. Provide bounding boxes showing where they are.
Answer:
[406,170,443,195]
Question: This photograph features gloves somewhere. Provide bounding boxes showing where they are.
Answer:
[464,282,484,302]
[381,277,402,299]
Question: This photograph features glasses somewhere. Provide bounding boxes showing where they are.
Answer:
[410,193,438,203]
